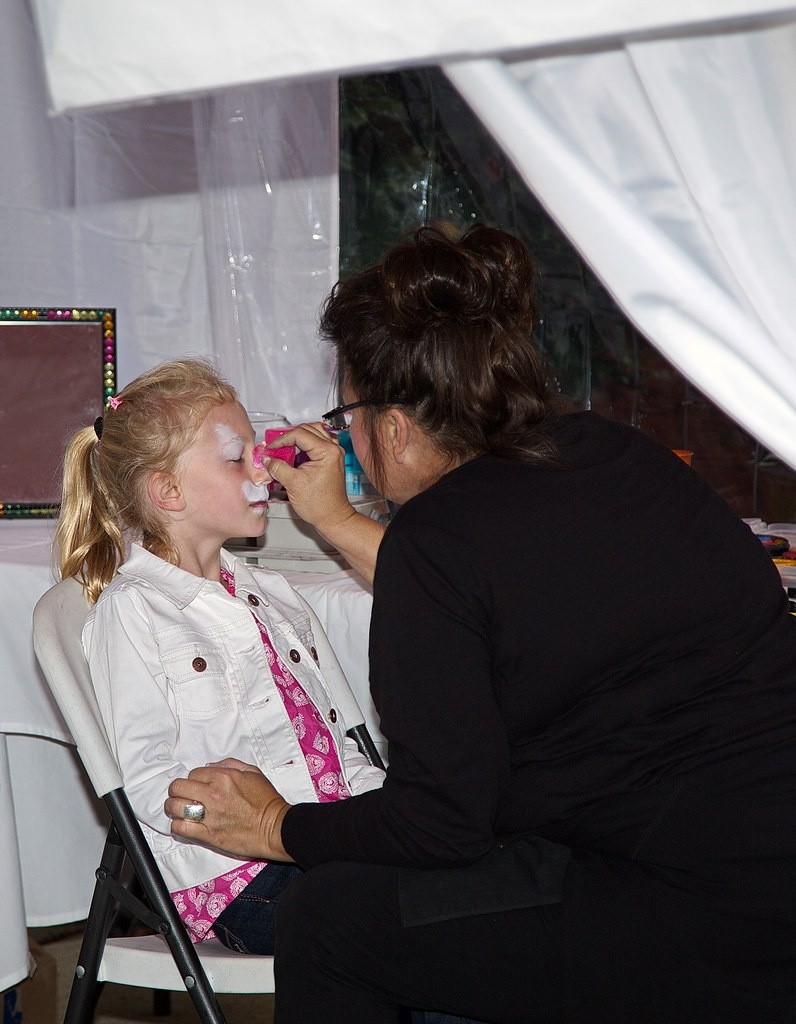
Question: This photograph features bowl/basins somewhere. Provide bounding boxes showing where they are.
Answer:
[245,410,291,450]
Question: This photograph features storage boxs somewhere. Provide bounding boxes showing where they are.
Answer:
[231,496,386,555]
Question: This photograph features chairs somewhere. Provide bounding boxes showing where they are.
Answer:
[32,573,275,1024]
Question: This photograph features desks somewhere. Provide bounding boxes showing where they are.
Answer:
[0,520,375,1024]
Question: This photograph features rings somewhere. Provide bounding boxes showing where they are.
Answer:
[183,800,207,824]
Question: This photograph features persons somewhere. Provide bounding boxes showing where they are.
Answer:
[50,360,388,956]
[163,223,796,1024]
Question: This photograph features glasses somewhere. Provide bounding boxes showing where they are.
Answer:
[322,393,416,431]
